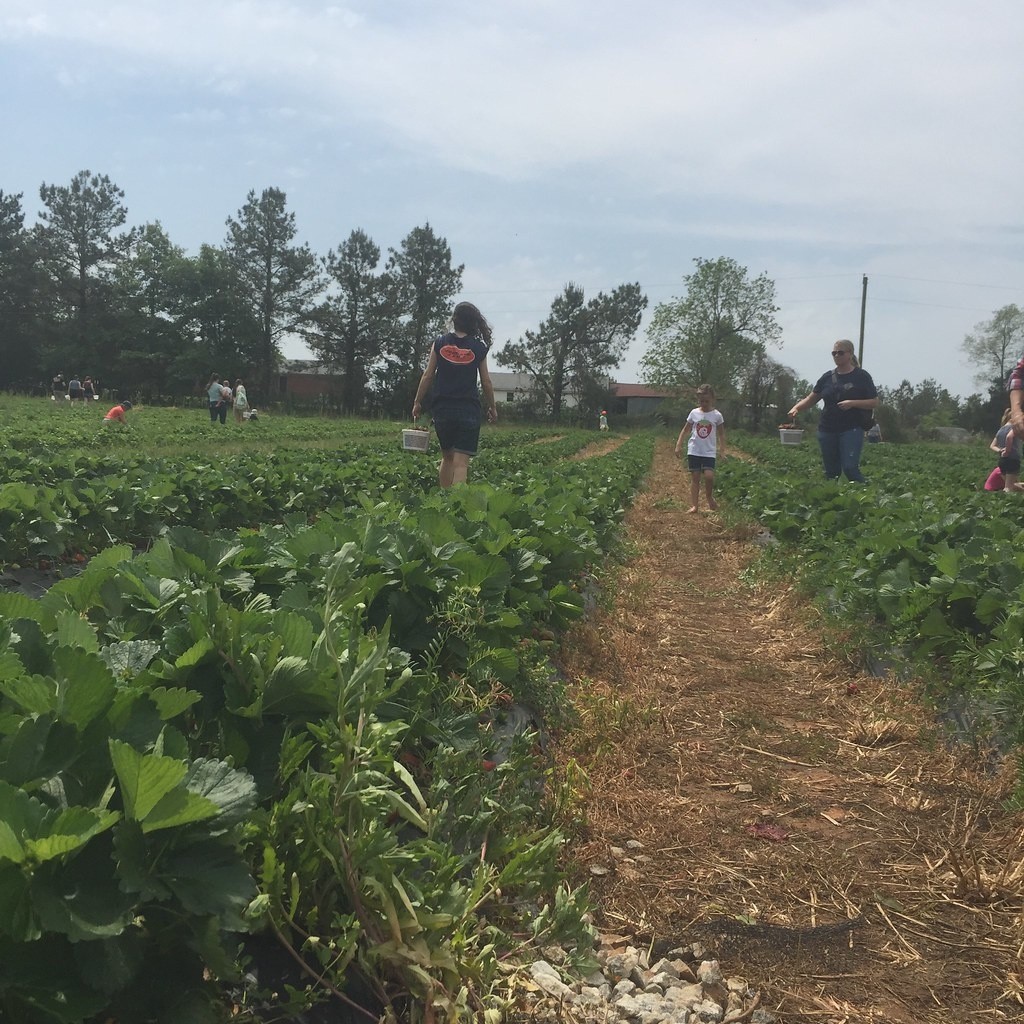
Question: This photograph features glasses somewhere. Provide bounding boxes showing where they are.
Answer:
[831,351,849,356]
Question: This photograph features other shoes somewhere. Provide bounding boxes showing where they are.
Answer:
[686,507,699,514]
[706,500,718,511]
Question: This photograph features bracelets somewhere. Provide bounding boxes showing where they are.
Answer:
[999,450,1001,453]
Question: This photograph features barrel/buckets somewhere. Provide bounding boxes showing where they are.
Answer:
[65,395,70,400]
[50,395,55,400]
[779,417,804,444]
[402,415,430,451]
[93,394,99,400]
[243,409,251,418]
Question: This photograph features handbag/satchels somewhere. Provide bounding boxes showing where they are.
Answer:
[854,408,875,431]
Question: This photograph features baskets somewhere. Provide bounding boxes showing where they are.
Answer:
[51,396,56,400]
[65,395,70,401]
[778,415,804,445]
[402,419,431,452]
[93,394,100,400]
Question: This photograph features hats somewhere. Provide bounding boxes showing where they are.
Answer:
[57,371,64,374]
[602,410,607,415]
[251,409,259,415]
[122,400,132,407]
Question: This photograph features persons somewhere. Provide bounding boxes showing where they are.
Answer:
[786,340,879,482]
[674,385,727,514]
[101,400,132,427]
[250,409,259,421]
[599,410,609,432]
[983,467,1024,491]
[206,372,229,424]
[989,408,1021,491]
[231,379,249,422]
[67,374,85,404]
[50,370,66,401]
[215,381,233,407]
[83,376,96,402]
[410,302,497,489]
[1004,352,1024,440]
[865,419,882,443]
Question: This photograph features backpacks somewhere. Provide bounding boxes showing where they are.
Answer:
[237,392,247,410]
